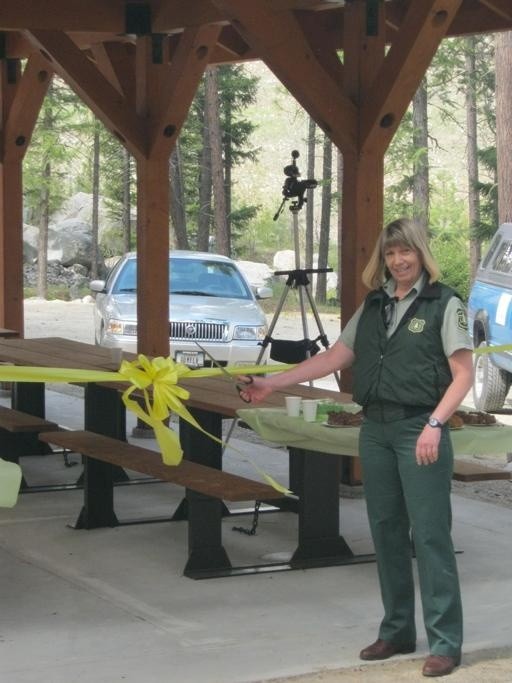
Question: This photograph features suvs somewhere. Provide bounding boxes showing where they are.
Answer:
[466,220,512,413]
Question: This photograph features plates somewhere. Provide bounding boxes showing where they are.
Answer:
[450,424,465,430]
[471,421,501,426]
[321,421,353,428]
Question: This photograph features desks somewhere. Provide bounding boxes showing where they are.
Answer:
[0,333,512,570]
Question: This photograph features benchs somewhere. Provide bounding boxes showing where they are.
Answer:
[0,389,62,493]
[39,426,285,583]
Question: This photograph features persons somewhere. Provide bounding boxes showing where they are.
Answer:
[232,215,474,677]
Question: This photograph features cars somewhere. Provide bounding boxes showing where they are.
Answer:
[89,247,272,382]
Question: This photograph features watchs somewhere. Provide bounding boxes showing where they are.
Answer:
[423,415,445,428]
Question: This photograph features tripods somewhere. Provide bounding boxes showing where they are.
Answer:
[216,211,379,468]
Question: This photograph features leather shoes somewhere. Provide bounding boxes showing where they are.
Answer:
[360,639,416,660]
[423,655,459,676]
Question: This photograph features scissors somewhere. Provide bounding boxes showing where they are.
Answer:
[187,340,253,403]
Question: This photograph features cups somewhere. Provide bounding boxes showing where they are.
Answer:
[285,395,302,417]
[110,347,122,363]
[301,400,319,420]
